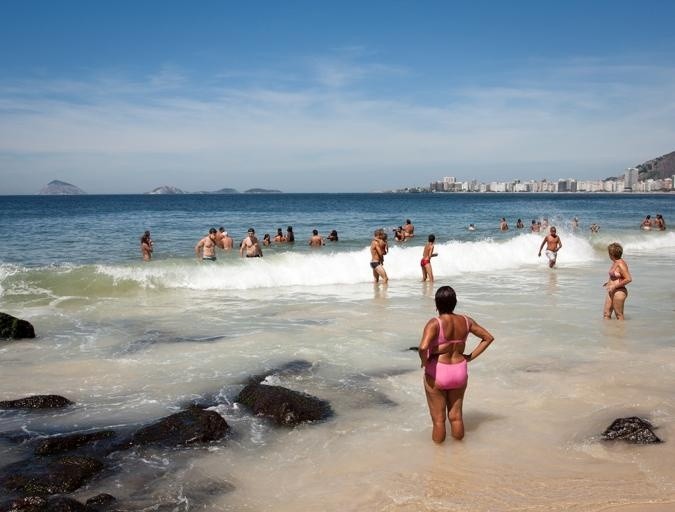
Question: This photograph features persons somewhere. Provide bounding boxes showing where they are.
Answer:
[640,214,666,232]
[194,218,415,262]
[139,231,154,261]
[370,229,388,284]
[602,242,633,321]
[468,215,602,237]
[538,226,563,268]
[418,285,494,445]
[420,234,438,282]
